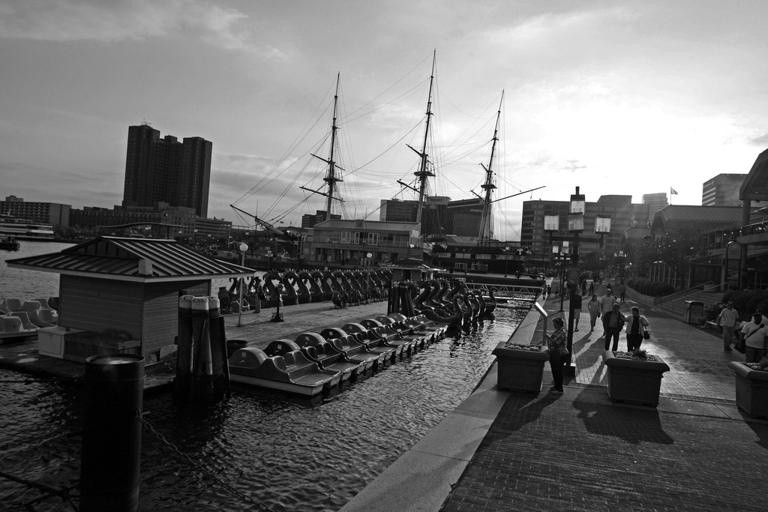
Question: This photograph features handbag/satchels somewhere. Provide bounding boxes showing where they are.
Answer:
[735,338,747,355]
[643,326,650,341]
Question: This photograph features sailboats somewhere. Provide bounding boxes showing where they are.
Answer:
[228,45,549,292]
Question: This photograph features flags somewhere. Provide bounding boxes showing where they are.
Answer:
[671,188,678,194]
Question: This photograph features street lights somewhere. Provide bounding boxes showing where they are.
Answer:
[543,184,610,376]
[552,240,571,312]
[365,251,372,303]
[237,241,248,328]
[613,250,626,303]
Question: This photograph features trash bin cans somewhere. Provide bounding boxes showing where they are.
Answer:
[682,300,704,324]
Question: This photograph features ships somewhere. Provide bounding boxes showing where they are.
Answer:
[0,211,55,242]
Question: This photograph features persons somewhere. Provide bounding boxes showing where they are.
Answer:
[574,289,582,332]
[751,309,768,326]
[607,284,613,295]
[621,308,649,352]
[717,301,739,352]
[588,282,594,296]
[741,313,768,363]
[600,289,615,336]
[618,282,625,303]
[587,294,601,332]
[545,319,570,395]
[582,281,587,296]
[603,303,625,352]
[547,285,551,297]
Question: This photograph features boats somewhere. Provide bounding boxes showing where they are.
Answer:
[212,266,496,398]
[0,291,58,344]
[495,297,533,311]
[0,236,20,252]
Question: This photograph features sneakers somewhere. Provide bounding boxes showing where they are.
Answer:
[550,387,563,393]
